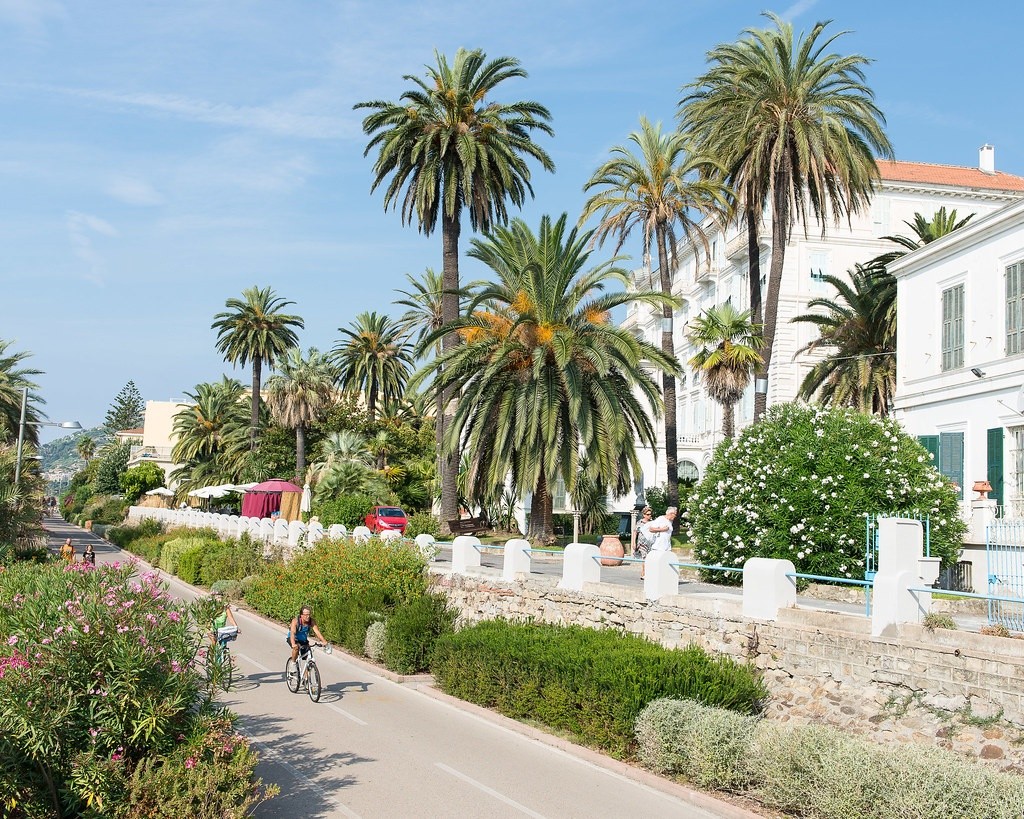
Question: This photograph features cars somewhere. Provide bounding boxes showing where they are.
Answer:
[364,505,409,535]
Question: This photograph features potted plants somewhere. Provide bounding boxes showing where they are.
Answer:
[599,514,625,567]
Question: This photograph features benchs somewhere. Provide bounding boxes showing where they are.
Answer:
[446,517,487,541]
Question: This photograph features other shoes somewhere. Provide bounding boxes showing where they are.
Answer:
[290,662,297,673]
[303,680,312,685]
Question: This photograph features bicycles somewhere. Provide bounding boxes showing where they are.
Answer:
[206,630,243,691]
[286,642,326,702]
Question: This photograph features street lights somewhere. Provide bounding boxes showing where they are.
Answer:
[13,387,83,501]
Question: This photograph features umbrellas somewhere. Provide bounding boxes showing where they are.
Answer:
[145,478,304,523]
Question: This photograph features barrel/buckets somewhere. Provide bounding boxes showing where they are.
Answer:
[600,534,624,566]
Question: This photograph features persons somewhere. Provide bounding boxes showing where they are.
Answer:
[631,507,661,581]
[649,506,677,552]
[204,592,243,670]
[287,605,328,695]
[58,538,76,566]
[82,544,96,567]
